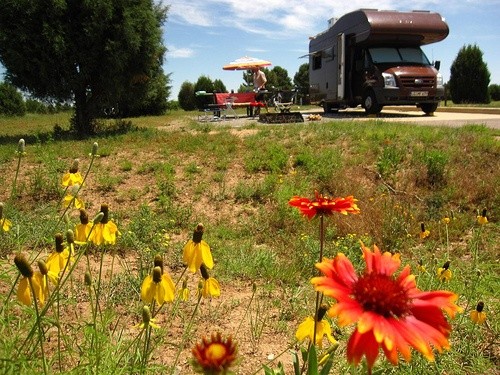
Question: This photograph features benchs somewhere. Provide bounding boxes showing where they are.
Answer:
[208,93,264,117]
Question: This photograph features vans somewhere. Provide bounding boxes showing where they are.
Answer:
[306,8,450,114]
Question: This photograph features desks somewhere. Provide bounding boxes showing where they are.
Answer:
[221,97,239,119]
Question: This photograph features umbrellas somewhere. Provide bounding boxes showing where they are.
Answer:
[223,55,272,92]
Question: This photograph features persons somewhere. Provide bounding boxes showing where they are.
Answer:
[252,66,268,92]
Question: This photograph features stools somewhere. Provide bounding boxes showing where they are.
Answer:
[276,102,293,113]
